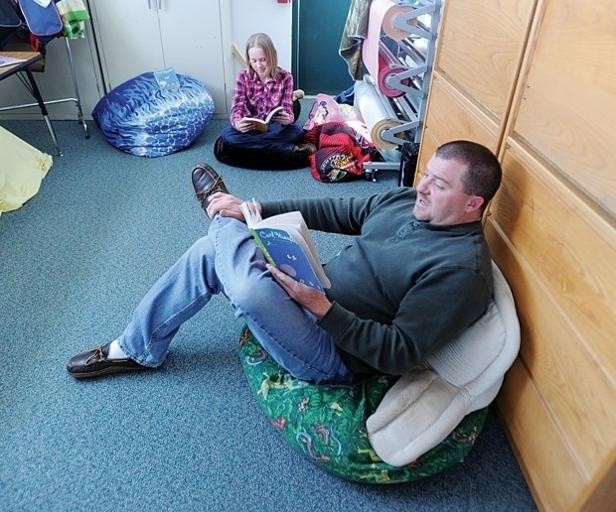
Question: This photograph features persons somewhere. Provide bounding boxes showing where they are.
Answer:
[66,139,501,385]
[221,33,318,155]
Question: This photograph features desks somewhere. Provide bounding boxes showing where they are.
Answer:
[0,50,65,158]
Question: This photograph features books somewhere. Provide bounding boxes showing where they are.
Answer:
[242,106,283,132]
[240,197,332,296]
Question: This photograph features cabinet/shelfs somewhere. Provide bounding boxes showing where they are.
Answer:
[411,0,539,236]
[479,1,616,512]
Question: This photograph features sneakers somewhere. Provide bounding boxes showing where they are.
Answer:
[292,142,317,157]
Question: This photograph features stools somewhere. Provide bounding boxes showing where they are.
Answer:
[90,70,216,159]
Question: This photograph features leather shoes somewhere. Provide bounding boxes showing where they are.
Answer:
[66,339,162,380]
[191,162,231,228]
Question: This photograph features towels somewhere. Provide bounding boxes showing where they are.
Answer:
[338,0,371,81]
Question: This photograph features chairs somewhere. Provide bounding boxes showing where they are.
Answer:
[237,259,521,486]
[214,100,314,171]
